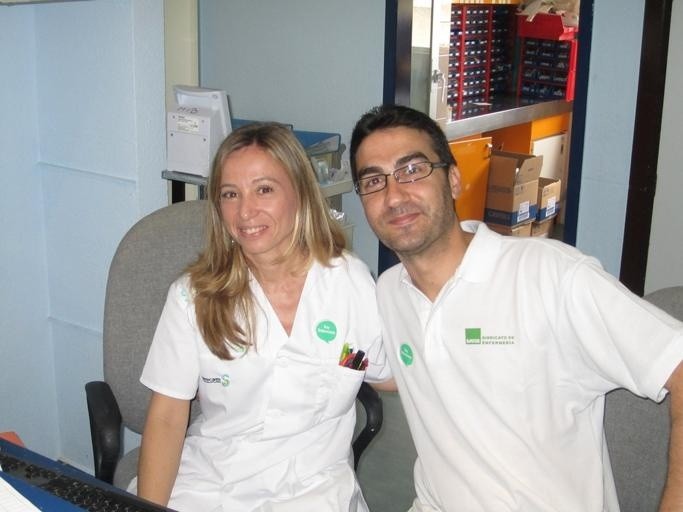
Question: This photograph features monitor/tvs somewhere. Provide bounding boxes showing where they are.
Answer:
[167,86,234,178]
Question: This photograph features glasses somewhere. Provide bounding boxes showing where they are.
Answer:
[355,161,449,195]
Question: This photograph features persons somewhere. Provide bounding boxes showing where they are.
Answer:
[350,103,683,512]
[125,124,398,512]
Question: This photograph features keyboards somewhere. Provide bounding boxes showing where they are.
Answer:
[0,452,169,512]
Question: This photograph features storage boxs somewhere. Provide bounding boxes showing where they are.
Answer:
[537,177,560,222]
[489,224,533,239]
[484,151,543,228]
[530,217,555,240]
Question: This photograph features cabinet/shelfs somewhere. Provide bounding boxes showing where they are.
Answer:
[434,0,581,242]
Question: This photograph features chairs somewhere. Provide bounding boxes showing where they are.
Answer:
[85,200,384,507]
[603,285,683,512]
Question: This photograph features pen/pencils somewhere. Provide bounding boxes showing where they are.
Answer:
[339,343,368,370]
